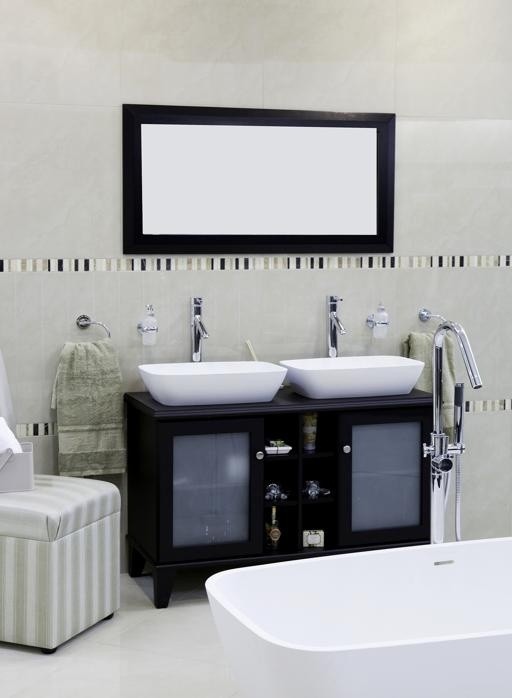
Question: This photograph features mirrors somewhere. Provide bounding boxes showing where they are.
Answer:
[122,103,395,254]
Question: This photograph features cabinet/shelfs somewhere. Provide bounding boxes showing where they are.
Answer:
[121,391,432,609]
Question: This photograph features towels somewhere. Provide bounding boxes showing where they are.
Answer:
[404,333,455,446]
[51,338,129,476]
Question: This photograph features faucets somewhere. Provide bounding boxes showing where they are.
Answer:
[190,296,209,362]
[324,294,346,358]
[421,318,484,547]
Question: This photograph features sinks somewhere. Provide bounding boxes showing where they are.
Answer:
[137,361,289,404]
[279,354,426,400]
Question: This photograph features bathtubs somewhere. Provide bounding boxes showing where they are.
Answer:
[205,535,510,697]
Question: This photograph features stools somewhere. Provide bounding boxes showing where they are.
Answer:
[0,474,121,654]
